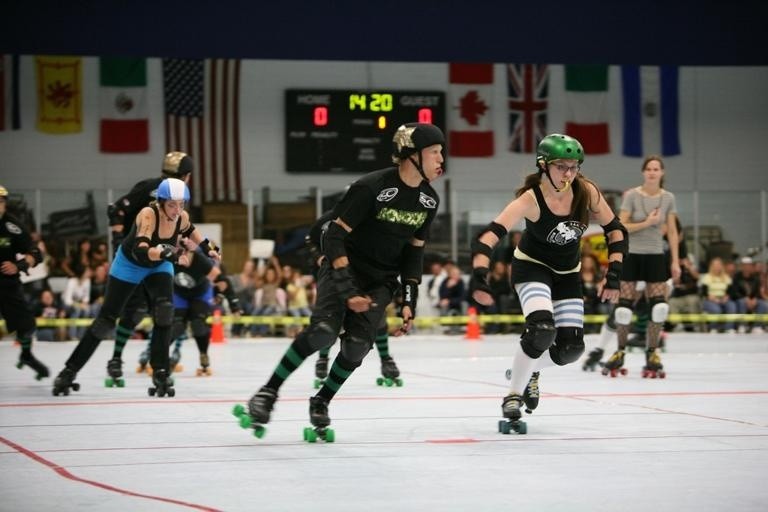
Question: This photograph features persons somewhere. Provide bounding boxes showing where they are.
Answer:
[2,151,768,378]
[470,133,629,418]
[248,122,445,427]
[53,175,223,390]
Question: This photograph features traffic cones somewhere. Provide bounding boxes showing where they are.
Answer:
[462,308,484,341]
[207,309,228,343]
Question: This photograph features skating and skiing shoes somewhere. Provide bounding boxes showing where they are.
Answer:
[499,368,539,434]
[304,397,336,442]
[196,354,212,376]
[314,358,328,388]
[136,349,182,397]
[232,385,276,438]
[104,359,124,387]
[17,349,48,379]
[53,369,78,395]
[377,356,403,386]
[584,331,665,379]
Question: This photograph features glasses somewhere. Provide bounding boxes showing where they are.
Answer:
[551,162,580,171]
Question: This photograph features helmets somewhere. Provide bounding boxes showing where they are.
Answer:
[162,151,191,176]
[156,178,190,203]
[536,133,584,172]
[390,122,444,166]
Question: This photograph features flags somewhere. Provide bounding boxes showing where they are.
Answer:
[0,54,22,133]
[162,57,243,206]
[561,64,612,157]
[33,55,84,135]
[620,65,682,159]
[504,63,551,156]
[98,57,149,154]
[446,63,496,159]
